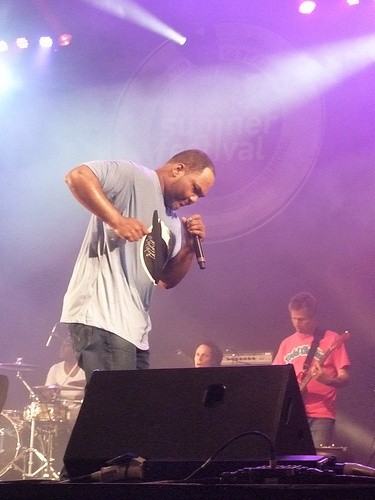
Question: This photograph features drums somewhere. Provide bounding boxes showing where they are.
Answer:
[0,413,41,478]
[24,401,67,427]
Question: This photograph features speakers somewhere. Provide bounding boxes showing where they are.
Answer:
[63,364,316,482]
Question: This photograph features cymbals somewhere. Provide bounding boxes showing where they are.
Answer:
[0,363,40,371]
[67,380,87,387]
[33,383,83,391]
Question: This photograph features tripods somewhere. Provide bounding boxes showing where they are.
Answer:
[0,371,60,480]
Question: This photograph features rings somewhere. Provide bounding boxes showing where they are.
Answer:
[313,373,316,376]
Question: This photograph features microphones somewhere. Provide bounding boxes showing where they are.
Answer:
[45,324,59,347]
[187,217,206,269]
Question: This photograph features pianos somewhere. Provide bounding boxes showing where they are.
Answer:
[221,352,273,365]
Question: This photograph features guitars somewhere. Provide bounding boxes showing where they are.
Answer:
[297,331,351,392]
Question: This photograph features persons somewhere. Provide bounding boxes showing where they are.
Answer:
[271,290,354,449]
[193,342,223,368]
[59,150,216,383]
[43,336,88,476]
